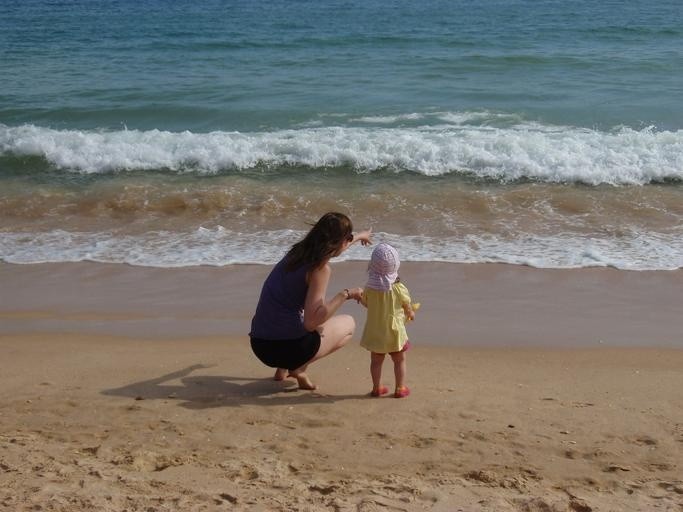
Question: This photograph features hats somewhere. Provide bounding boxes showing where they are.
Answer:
[367,243,400,291]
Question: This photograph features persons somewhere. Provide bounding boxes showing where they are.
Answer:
[358,243,416,398]
[248,211,374,391]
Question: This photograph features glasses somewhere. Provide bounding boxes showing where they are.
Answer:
[347,234,353,242]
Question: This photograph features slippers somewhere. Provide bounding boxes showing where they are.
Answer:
[395,386,410,397]
[371,386,388,397]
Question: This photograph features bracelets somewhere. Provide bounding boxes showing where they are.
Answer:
[344,288,351,300]
